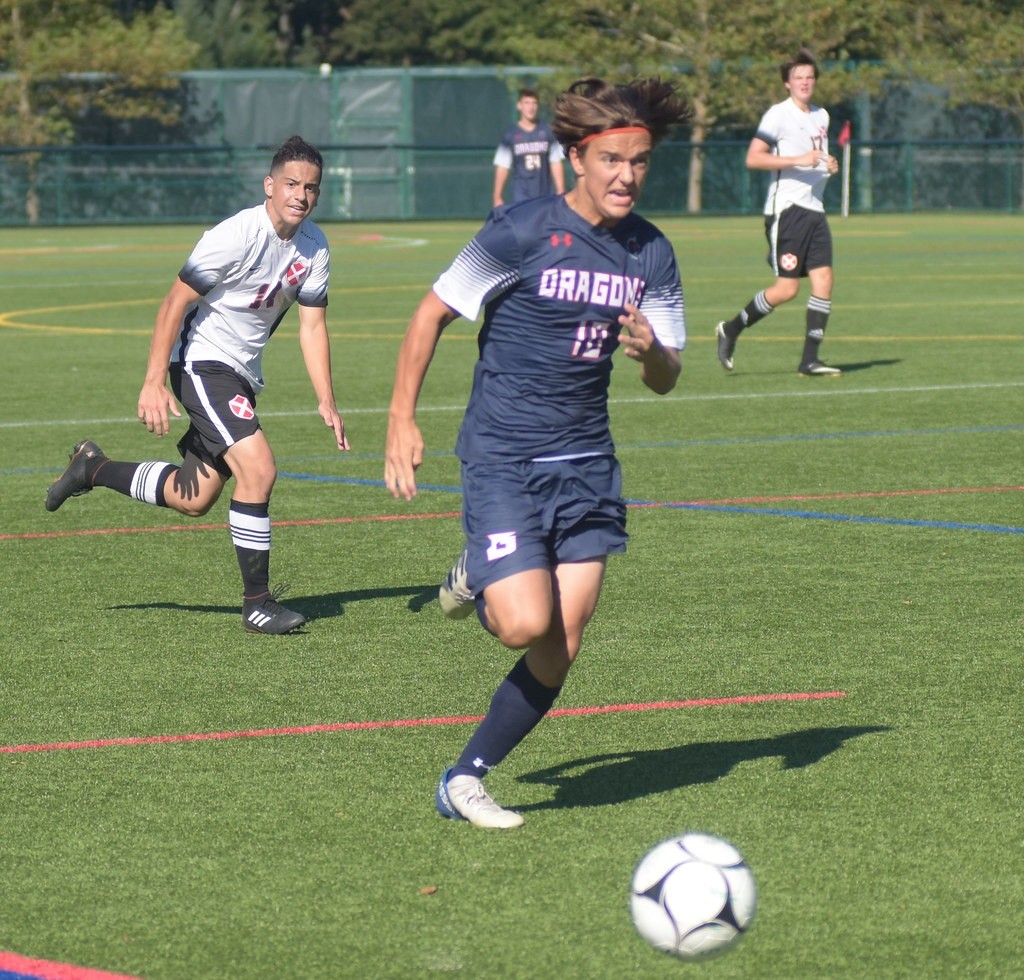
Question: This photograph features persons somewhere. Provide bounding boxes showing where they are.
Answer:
[714,49,840,377]
[491,89,565,208]
[384,77,683,829]
[45,135,350,633]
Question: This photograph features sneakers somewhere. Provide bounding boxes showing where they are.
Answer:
[713,321,738,371]
[438,548,477,622]
[434,767,526,829]
[43,439,104,512]
[797,360,842,379]
[241,595,306,635]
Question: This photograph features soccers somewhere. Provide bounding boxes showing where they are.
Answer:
[633,833,759,962]
